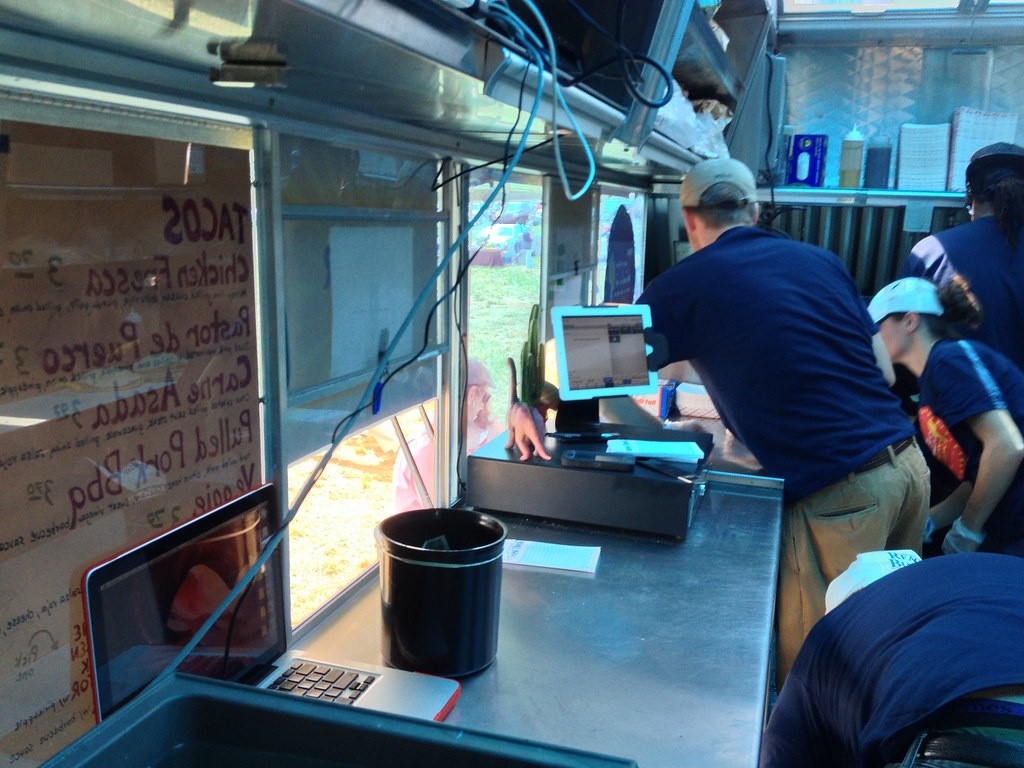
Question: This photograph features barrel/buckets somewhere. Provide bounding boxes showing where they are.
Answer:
[376,508,506,681]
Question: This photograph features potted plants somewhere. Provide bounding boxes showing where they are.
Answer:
[520,304,548,429]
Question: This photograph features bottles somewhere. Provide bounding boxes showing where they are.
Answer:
[842,121,892,191]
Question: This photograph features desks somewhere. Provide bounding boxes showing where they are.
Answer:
[186,365,788,766]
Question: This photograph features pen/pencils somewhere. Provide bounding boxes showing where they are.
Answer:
[560,436,620,443]
[372,328,388,415]
[545,432,614,438]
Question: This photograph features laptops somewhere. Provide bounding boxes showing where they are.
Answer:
[81,482,462,728]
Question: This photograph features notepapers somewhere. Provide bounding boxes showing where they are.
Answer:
[605,439,704,464]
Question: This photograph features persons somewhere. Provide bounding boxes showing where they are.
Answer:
[391,356,498,554]
[906,139,1024,442]
[864,274,1024,571]
[632,154,932,708]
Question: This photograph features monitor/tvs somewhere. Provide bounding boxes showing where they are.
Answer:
[551,304,658,401]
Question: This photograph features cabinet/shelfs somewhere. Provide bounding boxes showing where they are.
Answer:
[1,0,1024,305]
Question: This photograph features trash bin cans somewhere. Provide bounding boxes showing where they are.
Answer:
[374,506,509,678]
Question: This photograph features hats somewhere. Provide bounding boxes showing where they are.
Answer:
[867,277,942,324]
[970,142,1022,163]
[823,549,923,617]
[465,355,497,389]
[681,159,758,206]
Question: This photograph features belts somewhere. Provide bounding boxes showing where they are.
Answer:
[896,734,1024,767]
